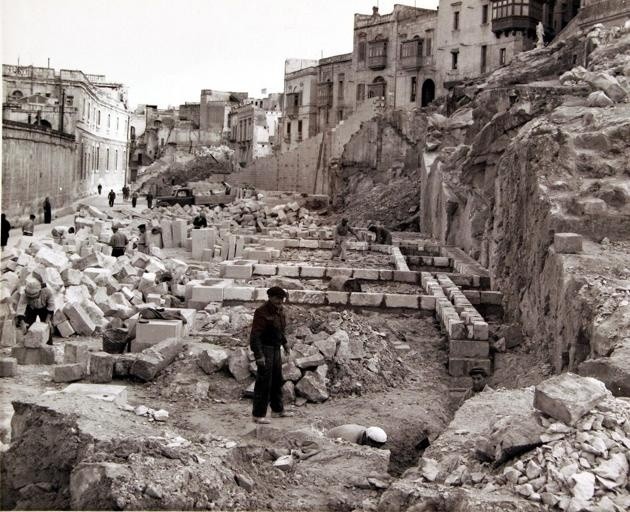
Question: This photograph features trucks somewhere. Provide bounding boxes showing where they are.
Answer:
[155,188,237,209]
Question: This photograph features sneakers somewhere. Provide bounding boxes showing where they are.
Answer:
[252,416,271,423]
[271,410,294,417]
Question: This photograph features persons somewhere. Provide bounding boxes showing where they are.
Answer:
[193,212,207,229]
[327,275,362,292]
[97,183,101,194]
[43,197,51,223]
[107,225,129,257]
[250,286,294,424]
[15,279,53,345]
[327,423,388,448]
[131,191,138,207]
[134,223,150,255]
[459,367,493,405]
[1,213,10,248]
[108,189,116,207]
[210,181,257,199]
[368,225,392,244]
[146,191,153,208]
[122,185,129,200]
[52,225,74,237]
[330,218,359,261]
[22,214,36,235]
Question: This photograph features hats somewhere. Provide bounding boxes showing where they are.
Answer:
[25,278,42,298]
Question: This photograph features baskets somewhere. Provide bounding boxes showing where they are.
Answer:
[103,329,128,354]
